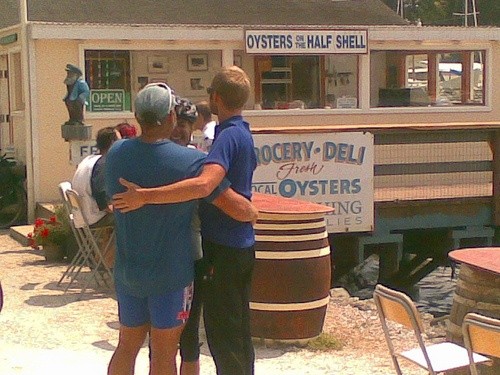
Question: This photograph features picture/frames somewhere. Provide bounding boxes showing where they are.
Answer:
[186,53,209,71]
[147,56,171,75]
[234,55,242,67]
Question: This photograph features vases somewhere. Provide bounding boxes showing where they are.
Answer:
[46,242,62,262]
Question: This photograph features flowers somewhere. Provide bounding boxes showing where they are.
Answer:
[27,217,56,247]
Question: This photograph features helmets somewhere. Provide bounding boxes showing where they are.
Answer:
[175,97,199,122]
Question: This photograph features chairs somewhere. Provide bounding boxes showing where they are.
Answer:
[58,182,115,300]
[374,285,491,375]
[462,312,500,375]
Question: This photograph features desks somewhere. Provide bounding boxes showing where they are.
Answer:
[449,247,500,281]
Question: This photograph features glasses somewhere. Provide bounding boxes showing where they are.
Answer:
[207,88,215,94]
[144,82,172,114]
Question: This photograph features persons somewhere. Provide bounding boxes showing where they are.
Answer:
[110,65,257,375]
[62,63,91,125]
[68,125,122,229]
[168,97,208,375]
[193,100,216,151]
[102,81,260,375]
